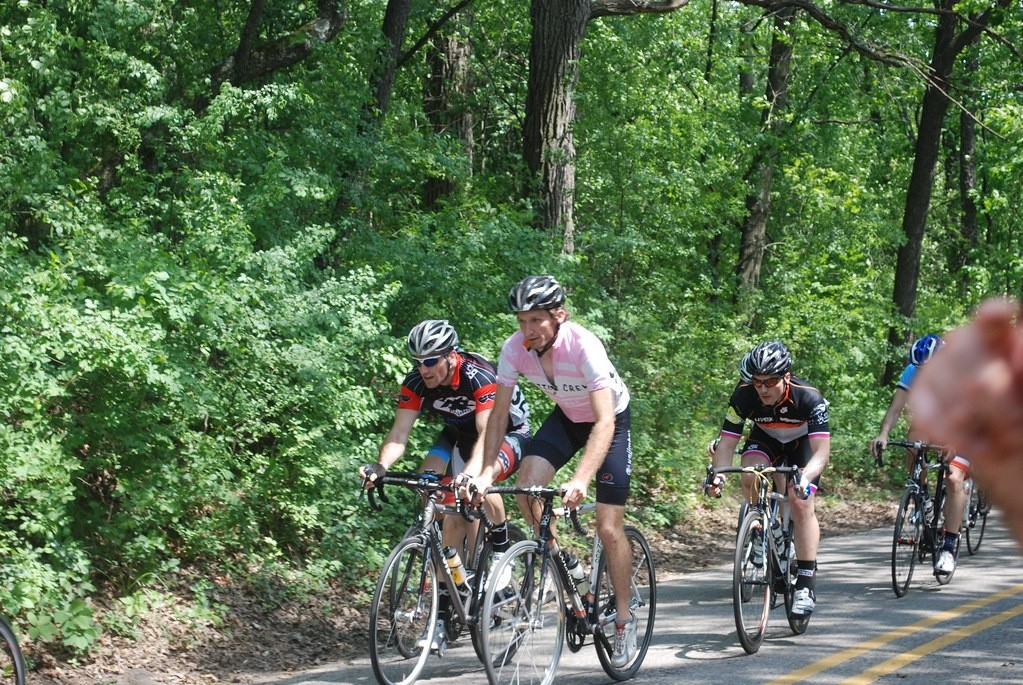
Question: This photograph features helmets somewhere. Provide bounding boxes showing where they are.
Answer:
[746,341,790,375]
[408,320,458,357]
[740,354,752,383]
[910,334,944,368]
[508,276,565,314]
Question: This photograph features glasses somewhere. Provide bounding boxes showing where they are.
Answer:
[751,375,784,387]
[414,352,448,367]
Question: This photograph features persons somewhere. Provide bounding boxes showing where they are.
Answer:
[705,341,830,620]
[869,334,970,574]
[909,299,1023,557]
[358,319,531,650]
[466,275,637,668]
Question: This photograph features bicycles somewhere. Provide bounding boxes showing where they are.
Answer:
[358,468,535,684]
[468,485,658,685]
[873,441,986,597]
[704,464,818,653]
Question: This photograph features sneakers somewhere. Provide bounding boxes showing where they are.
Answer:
[934,549,954,573]
[978,490,990,515]
[415,619,447,649]
[910,502,934,524]
[533,555,584,604]
[749,526,768,568]
[790,584,816,618]
[611,610,637,666]
[483,550,515,592]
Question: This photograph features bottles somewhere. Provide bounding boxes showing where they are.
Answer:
[780,541,796,573]
[443,545,467,584]
[772,519,785,555]
[925,495,934,524]
[561,551,589,597]
